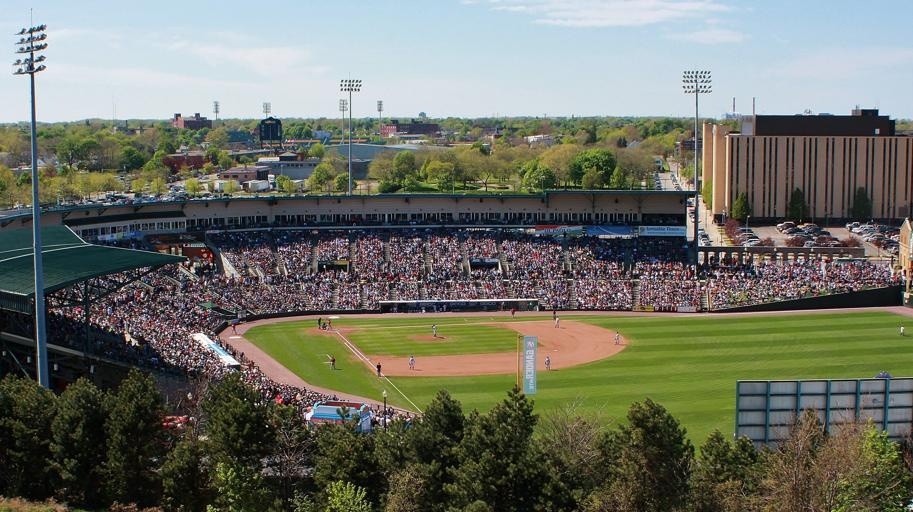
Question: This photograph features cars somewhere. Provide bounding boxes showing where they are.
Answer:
[668,171,901,256]
[84,192,266,209]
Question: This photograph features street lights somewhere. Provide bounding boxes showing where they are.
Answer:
[13,6,51,388]
[683,68,714,279]
[340,72,363,198]
[381,389,389,435]
[745,215,751,247]
